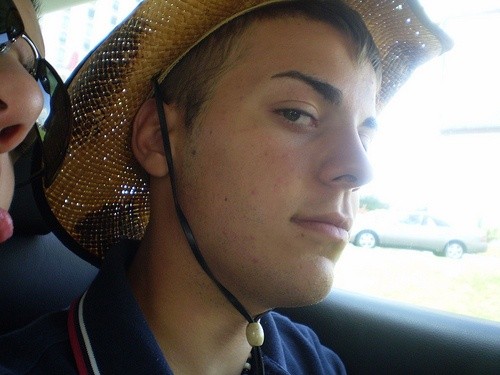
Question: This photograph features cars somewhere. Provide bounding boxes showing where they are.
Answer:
[346,220,488,260]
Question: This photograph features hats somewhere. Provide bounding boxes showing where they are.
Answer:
[29,0,452,270]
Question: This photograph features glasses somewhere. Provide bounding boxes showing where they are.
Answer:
[0,0,72,190]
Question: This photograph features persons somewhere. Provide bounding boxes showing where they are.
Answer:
[0,0,47,243]
[1,0,453,375]
